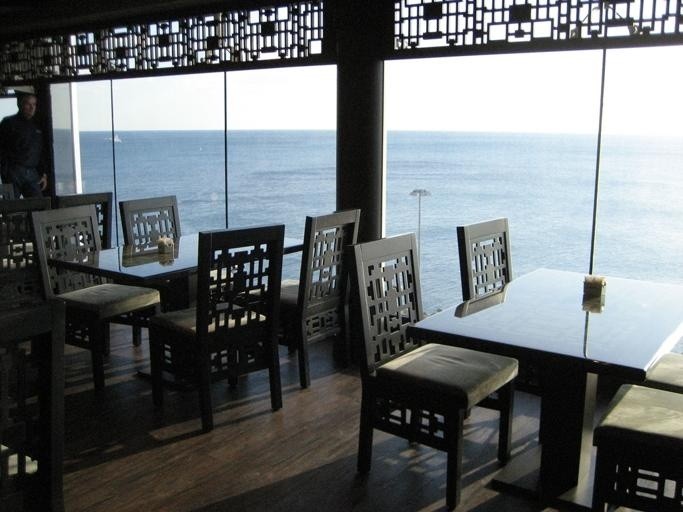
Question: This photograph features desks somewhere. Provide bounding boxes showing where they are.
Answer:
[61,237,323,362]
[407,265,682,511]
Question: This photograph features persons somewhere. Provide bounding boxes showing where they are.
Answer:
[0,91,50,201]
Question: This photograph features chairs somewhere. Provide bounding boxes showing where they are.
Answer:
[32,205,161,392]
[350,231,519,510]
[117,194,190,345]
[150,223,287,434]
[0,180,116,283]
[457,218,544,445]
[647,348,683,390]
[592,384,682,509]
[240,204,364,388]
